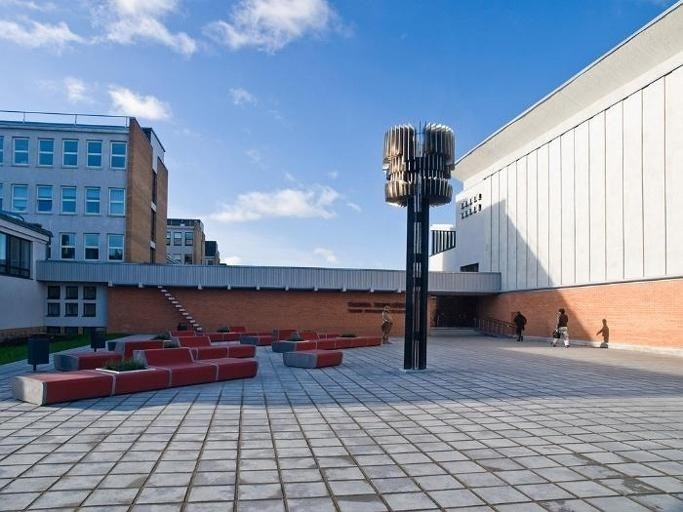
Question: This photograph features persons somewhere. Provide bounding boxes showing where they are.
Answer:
[550,308,569,348]
[379,305,394,345]
[513,311,527,341]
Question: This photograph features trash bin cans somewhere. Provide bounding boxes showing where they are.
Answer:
[177,322,187,331]
[27,333,49,365]
[90,328,106,348]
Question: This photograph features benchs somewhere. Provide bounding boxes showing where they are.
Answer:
[13,327,258,406]
[240,326,382,370]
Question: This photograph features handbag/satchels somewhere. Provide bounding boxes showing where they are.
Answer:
[553,331,560,338]
